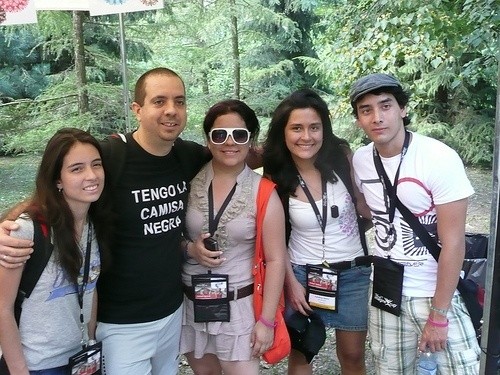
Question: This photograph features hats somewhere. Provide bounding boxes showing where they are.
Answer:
[287,310,326,363]
[349,73,399,102]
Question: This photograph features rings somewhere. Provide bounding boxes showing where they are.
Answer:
[2,255,7,261]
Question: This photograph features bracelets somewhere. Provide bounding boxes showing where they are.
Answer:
[184,240,199,265]
[259,315,278,328]
[427,316,449,327]
[431,306,448,314]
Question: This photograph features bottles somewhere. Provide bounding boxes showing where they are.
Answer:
[416,344,438,375]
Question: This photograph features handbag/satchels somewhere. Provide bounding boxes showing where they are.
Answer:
[252,178,292,365]
[370,255,404,318]
[457,278,483,330]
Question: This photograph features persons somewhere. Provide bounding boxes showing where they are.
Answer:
[350,73,481,375]
[0,127,105,374]
[0,68,264,374]
[181,99,286,375]
[264,90,371,375]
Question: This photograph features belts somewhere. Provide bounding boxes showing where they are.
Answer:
[323,256,369,272]
[182,282,254,303]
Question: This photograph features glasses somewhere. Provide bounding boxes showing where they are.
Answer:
[208,128,252,144]
